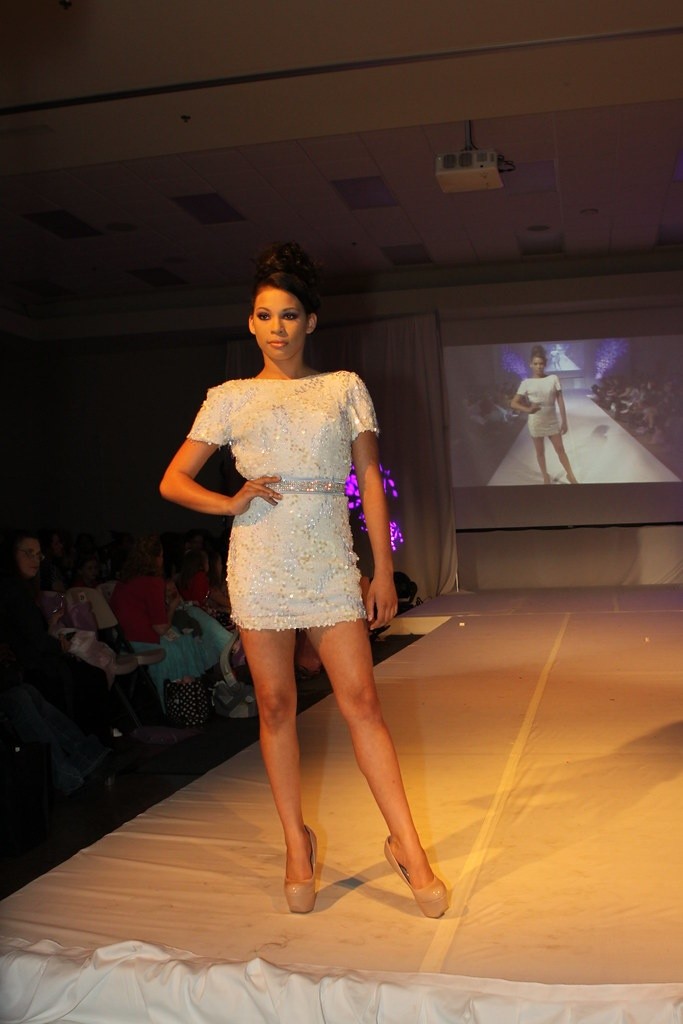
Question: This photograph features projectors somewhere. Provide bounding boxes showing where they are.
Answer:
[434,148,517,192]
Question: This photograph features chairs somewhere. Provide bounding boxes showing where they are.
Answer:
[66,586,164,745]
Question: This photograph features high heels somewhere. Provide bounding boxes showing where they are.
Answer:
[383,836,449,919]
[284,825,317,914]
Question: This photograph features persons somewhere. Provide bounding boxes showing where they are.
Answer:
[591,370,682,440]
[157,271,450,924]
[2,648,117,809]
[454,380,516,469]
[0,524,260,745]
[510,345,577,485]
